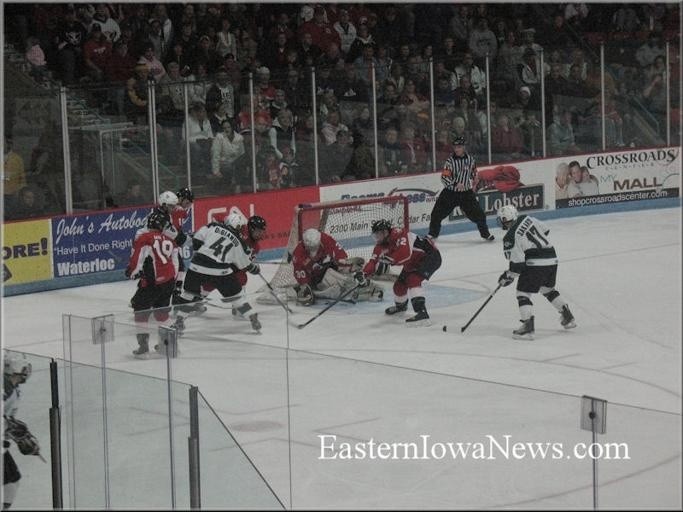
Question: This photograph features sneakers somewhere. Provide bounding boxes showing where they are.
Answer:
[512,316,534,334]
[231,304,243,318]
[406,297,429,322]
[156,324,176,348]
[425,233,435,239]
[175,316,186,330]
[559,305,574,325]
[249,312,260,330]
[386,299,409,315]
[482,233,494,241]
[132,333,148,355]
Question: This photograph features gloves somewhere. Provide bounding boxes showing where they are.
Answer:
[355,272,368,286]
[377,259,392,274]
[248,263,259,274]
[315,281,327,290]
[10,418,26,436]
[339,258,348,273]
[17,433,38,455]
[454,183,464,192]
[472,179,480,191]
[499,271,514,287]
[182,236,193,247]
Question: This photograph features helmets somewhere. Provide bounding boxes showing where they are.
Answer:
[5,354,30,376]
[452,138,468,145]
[248,215,265,229]
[176,188,194,204]
[371,220,390,234]
[498,204,518,228]
[158,191,177,208]
[147,212,166,231]
[226,213,242,235]
[303,229,322,251]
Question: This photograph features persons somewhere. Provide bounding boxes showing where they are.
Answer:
[209,119,245,179]
[201,212,267,299]
[171,42,194,77]
[173,4,199,24]
[270,89,299,120]
[257,147,315,192]
[553,161,574,198]
[170,212,262,335]
[137,44,167,83]
[270,107,296,158]
[281,48,303,84]
[216,17,237,62]
[127,191,187,312]
[119,6,149,41]
[326,128,359,183]
[126,63,173,119]
[351,99,447,179]
[323,111,349,145]
[109,41,137,88]
[363,218,443,327]
[581,165,596,186]
[173,22,199,50]
[206,4,234,29]
[113,23,139,56]
[89,5,121,43]
[160,62,193,115]
[297,2,682,99]
[297,113,324,148]
[3,133,26,221]
[206,67,240,134]
[318,91,340,128]
[148,4,172,44]
[125,211,176,356]
[568,161,597,196]
[266,10,292,43]
[216,52,242,87]
[236,92,271,145]
[2,350,41,511]
[447,99,682,162]
[247,115,271,159]
[496,204,578,340]
[181,101,214,166]
[191,35,219,73]
[424,138,495,241]
[171,187,194,305]
[143,19,172,66]
[249,65,277,109]
[83,23,113,80]
[9,186,45,220]
[187,61,212,104]
[269,32,289,70]
[291,228,384,303]
